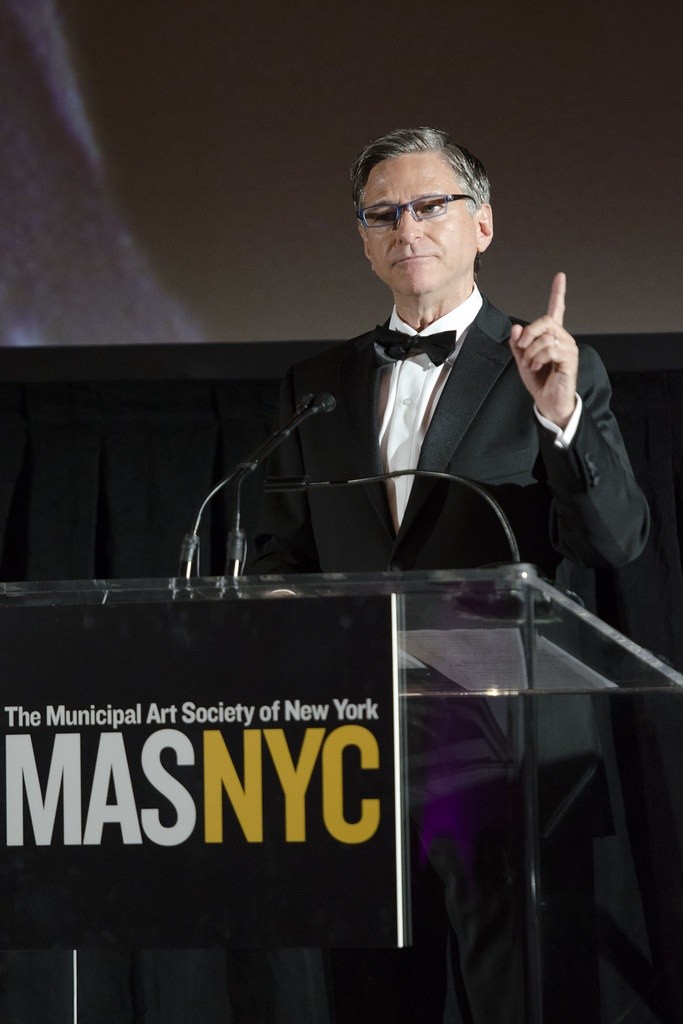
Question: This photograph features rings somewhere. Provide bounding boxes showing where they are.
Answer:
[553,336,559,347]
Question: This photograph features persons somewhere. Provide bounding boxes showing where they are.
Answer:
[249,126,650,1023]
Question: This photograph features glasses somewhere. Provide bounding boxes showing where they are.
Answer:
[357,194,476,227]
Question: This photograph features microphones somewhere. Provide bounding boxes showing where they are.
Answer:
[169,389,338,599]
[264,468,538,582]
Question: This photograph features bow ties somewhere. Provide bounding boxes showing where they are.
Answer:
[374,323,456,365]
